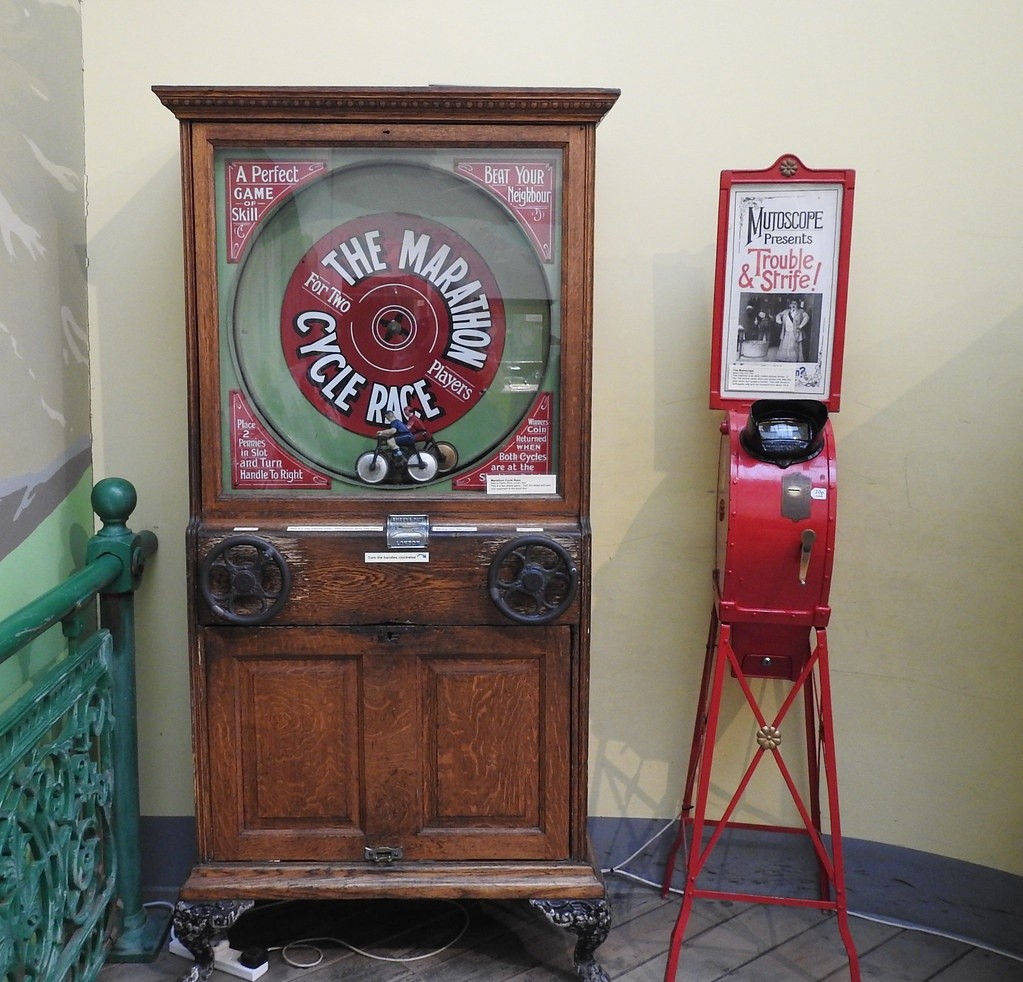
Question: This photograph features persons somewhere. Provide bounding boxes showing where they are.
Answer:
[775,300,810,362]
[404,406,432,442]
[377,411,415,457]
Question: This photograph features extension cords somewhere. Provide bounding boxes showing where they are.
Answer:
[169,937,269,982]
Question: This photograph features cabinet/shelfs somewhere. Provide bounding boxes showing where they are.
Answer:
[153,85,621,982]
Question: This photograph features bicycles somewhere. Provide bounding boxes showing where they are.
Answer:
[356,431,438,488]
[382,435,459,477]
